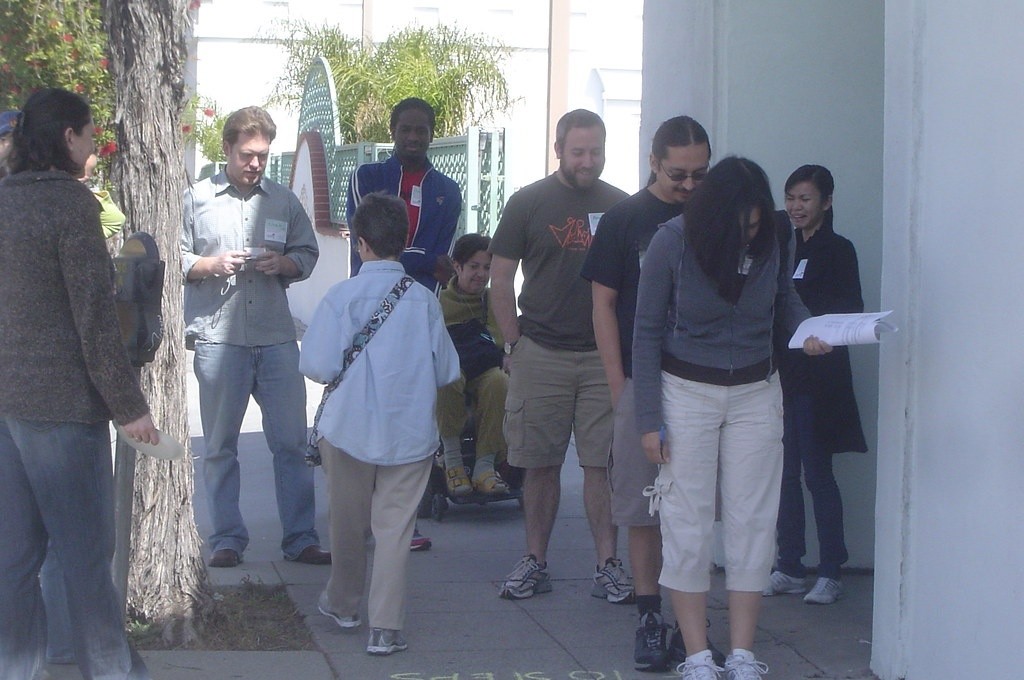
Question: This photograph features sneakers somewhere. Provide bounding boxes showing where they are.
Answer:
[669,618,727,668]
[633,608,674,670]
[724,654,769,680]
[677,653,724,680]
[803,576,844,604]
[367,627,408,653]
[761,571,806,596]
[409,528,432,551]
[319,603,362,628]
[499,555,553,600]
[590,557,637,604]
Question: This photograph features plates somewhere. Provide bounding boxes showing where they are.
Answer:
[117,424,186,461]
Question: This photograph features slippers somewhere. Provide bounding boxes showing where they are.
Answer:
[470,470,509,496]
[447,467,473,496]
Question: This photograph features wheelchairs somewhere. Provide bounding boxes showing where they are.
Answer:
[413,392,525,522]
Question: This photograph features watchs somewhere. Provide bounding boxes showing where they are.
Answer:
[504,341,517,355]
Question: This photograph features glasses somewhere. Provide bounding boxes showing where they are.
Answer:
[658,159,710,180]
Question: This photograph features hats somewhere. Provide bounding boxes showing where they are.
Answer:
[0,111,23,137]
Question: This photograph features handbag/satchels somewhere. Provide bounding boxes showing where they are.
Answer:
[305,386,332,466]
[441,319,502,370]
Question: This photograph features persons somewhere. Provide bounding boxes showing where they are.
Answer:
[0,87,161,680]
[762,165,869,604]
[633,156,833,680]
[301,193,461,655]
[487,109,631,602]
[181,107,331,569]
[581,115,726,669]
[345,99,462,550]
[436,234,511,498]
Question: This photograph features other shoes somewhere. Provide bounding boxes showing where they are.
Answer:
[282,545,332,564]
[209,549,243,567]
